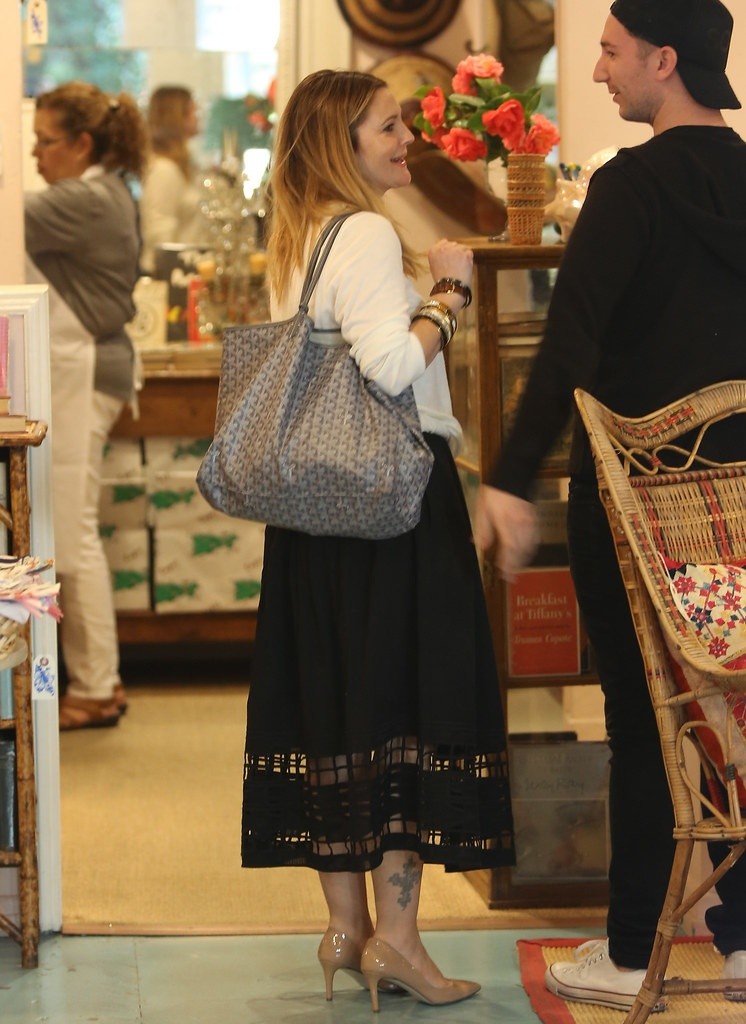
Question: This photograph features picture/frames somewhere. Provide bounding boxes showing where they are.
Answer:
[0,283,62,935]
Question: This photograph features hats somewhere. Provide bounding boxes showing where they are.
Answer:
[609,0,741,109]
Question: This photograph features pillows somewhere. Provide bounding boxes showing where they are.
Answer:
[659,557,746,810]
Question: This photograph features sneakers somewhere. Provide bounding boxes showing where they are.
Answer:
[544,936,669,1013]
[714,946,746,1001]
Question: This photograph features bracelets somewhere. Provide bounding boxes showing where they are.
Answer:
[430,278,473,309]
[412,300,458,350]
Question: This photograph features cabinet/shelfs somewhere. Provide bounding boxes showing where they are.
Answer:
[110,378,267,643]
[466,237,570,484]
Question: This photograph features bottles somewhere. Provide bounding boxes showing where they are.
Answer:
[166,252,272,346]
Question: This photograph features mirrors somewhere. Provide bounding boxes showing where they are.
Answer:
[21,1,280,276]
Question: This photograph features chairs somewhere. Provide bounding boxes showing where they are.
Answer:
[574,380,746,1024]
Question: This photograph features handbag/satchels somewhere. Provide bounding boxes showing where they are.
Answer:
[196,214,434,541]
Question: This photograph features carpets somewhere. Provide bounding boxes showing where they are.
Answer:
[60,685,607,936]
[516,936,746,1024]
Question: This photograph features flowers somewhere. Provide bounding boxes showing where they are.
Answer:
[412,53,561,167]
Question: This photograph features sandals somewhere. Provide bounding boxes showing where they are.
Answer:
[59,683,127,731]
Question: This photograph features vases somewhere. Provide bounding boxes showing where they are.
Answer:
[507,153,545,245]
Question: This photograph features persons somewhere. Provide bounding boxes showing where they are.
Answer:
[24,83,150,732]
[472,1,746,1014]
[136,87,233,278]
[240,70,514,1012]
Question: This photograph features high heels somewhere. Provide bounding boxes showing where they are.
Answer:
[360,936,481,1012]
[318,927,408,1001]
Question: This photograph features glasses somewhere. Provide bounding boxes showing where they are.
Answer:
[37,136,67,152]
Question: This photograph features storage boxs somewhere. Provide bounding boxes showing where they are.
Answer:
[143,437,265,616]
[94,441,150,612]
